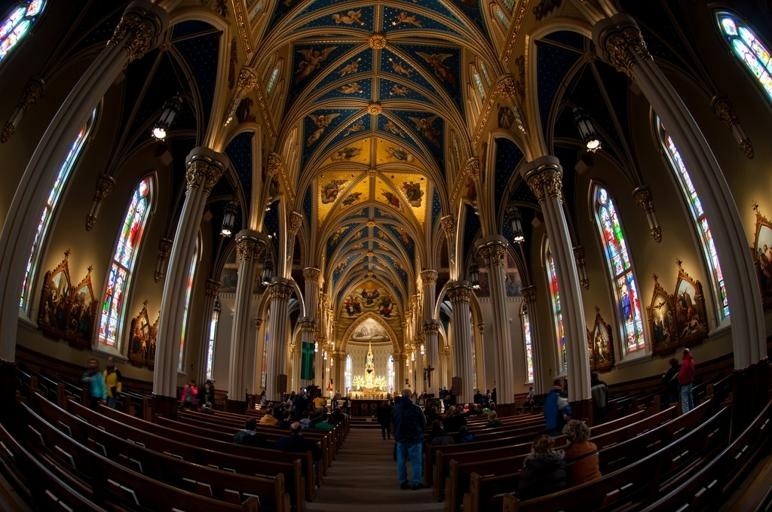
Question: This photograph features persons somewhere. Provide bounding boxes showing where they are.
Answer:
[83,362,351,487]
[758,248,771,279]
[761,244,771,263]
[292,10,456,208]
[379,347,697,488]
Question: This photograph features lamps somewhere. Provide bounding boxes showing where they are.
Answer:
[571,104,604,156]
[258,254,273,286]
[150,50,212,143]
[218,178,242,240]
[468,252,481,289]
[508,205,528,245]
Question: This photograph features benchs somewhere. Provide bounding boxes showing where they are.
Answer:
[416,350,770,509]
[0,347,353,510]
